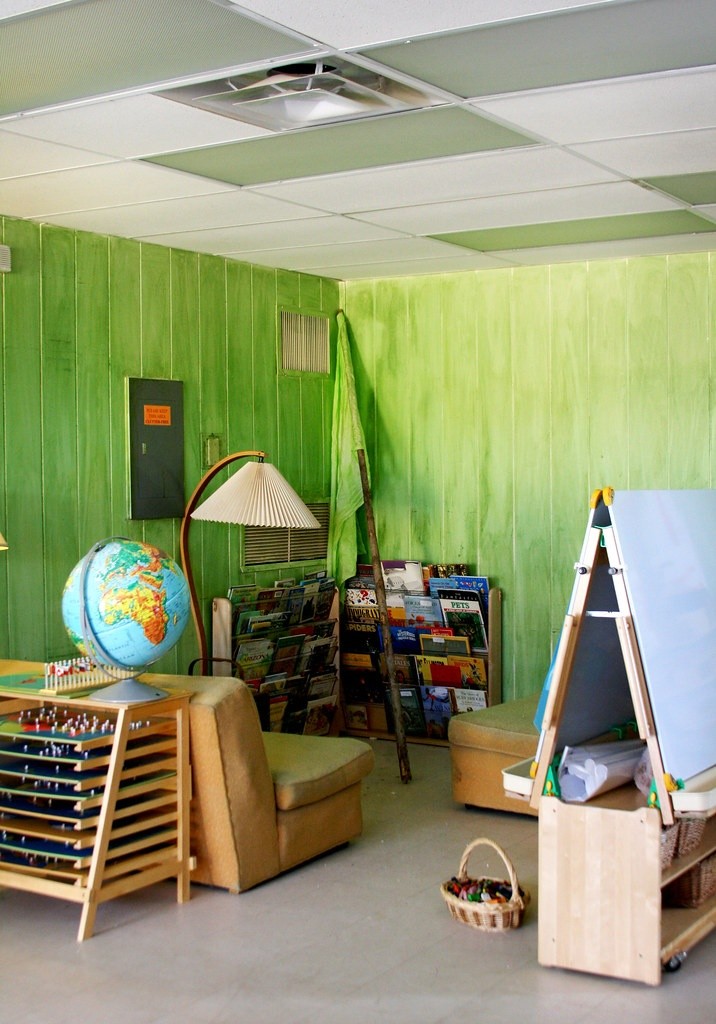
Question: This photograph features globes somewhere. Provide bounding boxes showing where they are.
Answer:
[60,536,191,704]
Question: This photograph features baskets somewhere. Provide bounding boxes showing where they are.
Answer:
[438,837,531,933]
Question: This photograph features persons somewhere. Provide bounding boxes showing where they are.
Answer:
[424,688,435,711]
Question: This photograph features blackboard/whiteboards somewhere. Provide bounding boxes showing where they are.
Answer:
[501,486,716,825]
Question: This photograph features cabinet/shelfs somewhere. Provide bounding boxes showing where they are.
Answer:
[210,587,503,746]
[537,782,716,987]
[1,673,194,941]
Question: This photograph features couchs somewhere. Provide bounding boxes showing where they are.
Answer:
[447,693,537,821]
[136,673,374,893]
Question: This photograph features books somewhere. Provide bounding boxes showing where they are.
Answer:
[227,569,339,736]
[341,560,489,741]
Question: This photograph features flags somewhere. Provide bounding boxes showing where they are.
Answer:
[328,312,372,608]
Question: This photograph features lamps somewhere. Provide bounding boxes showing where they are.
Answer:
[180,451,321,672]
